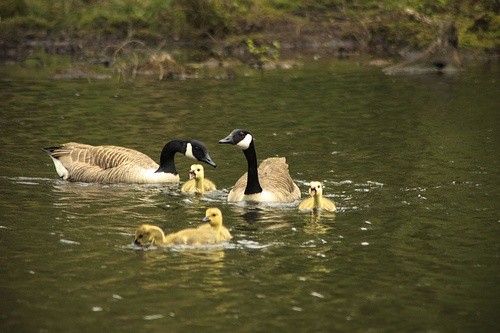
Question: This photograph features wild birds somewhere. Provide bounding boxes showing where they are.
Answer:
[219,128,301,208]
[298,181,336,212]
[133,225,165,248]
[166,207,233,245]
[180,163,215,192]
[41,139,218,184]
